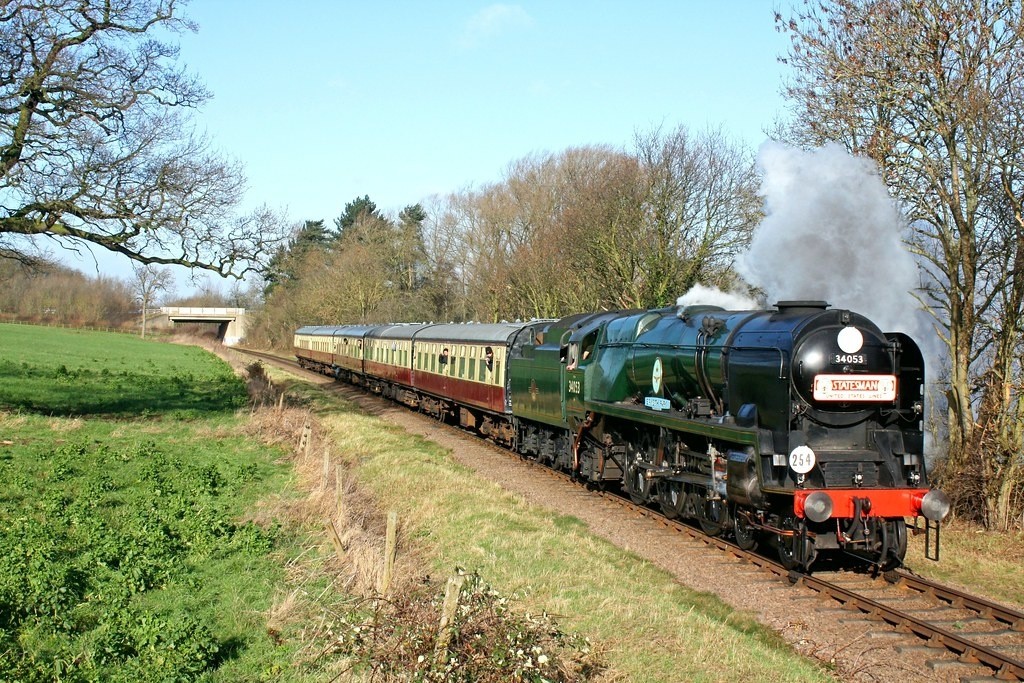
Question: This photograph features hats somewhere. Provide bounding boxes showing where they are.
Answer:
[486,347,492,354]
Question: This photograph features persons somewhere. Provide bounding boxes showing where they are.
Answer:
[344,338,348,345]
[485,347,493,372]
[566,338,594,370]
[358,340,361,349]
[440,348,448,364]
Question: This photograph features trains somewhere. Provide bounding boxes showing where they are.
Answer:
[293,300,951,571]
